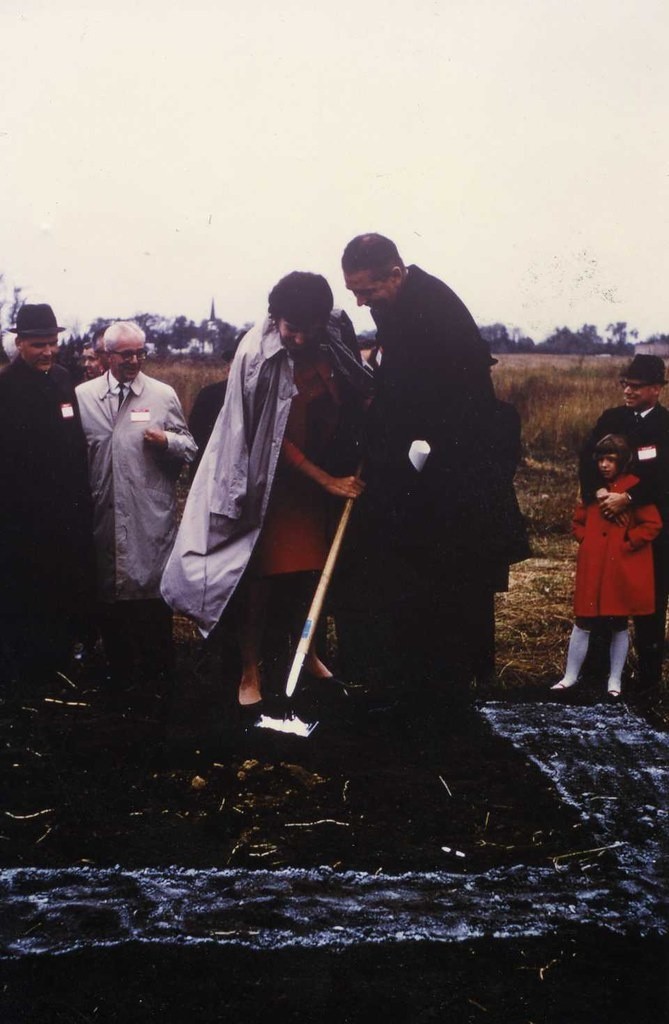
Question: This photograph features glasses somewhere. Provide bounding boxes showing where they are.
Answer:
[111,350,147,361]
[620,379,654,391]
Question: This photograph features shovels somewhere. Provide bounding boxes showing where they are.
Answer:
[253,458,364,739]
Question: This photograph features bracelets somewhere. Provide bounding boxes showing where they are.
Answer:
[627,493,631,501]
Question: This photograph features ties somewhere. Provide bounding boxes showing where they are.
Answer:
[118,383,125,411]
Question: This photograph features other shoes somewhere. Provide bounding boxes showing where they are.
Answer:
[237,681,263,714]
[605,678,623,704]
[547,681,578,694]
[304,666,340,689]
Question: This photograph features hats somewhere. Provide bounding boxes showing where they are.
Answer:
[8,304,67,335]
[620,354,669,384]
[486,342,498,365]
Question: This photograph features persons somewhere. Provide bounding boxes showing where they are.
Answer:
[541,433,663,698]
[578,356,669,697]
[0,241,533,722]
